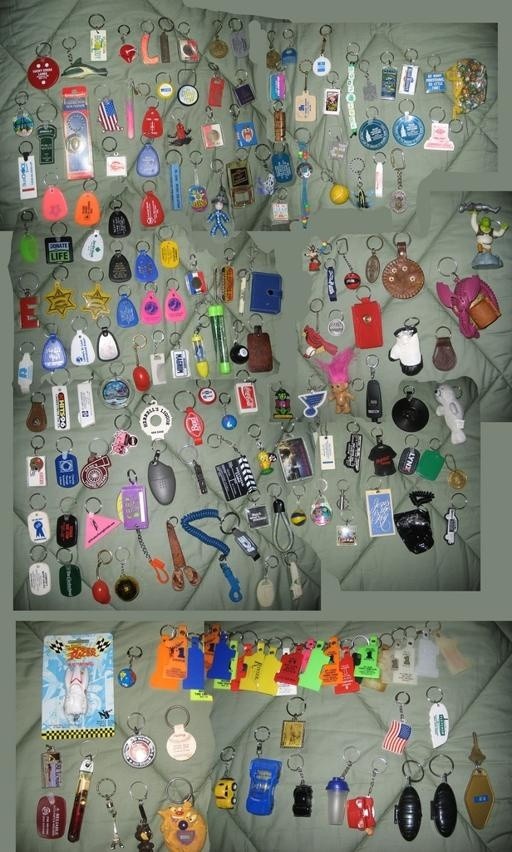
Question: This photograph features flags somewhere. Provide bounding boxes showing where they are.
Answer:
[381,720,411,754]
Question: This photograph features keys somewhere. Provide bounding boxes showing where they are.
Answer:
[467,729,484,767]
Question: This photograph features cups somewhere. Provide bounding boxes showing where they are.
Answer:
[325,776,349,825]
